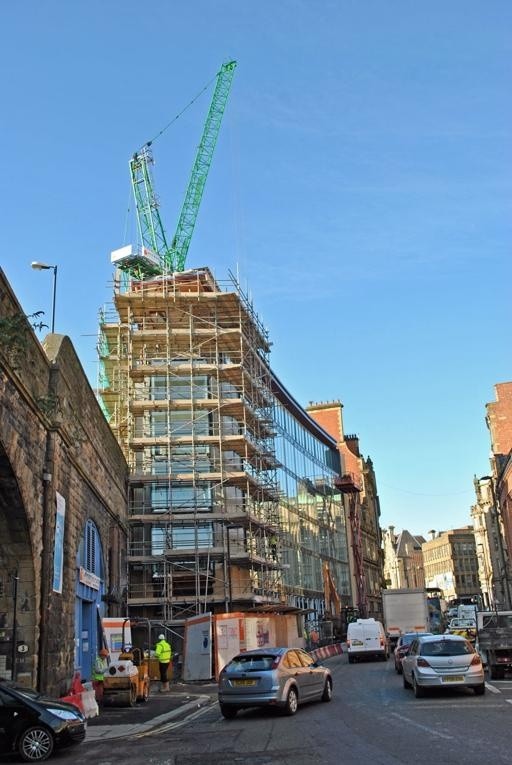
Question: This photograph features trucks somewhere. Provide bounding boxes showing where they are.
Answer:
[475,610,511,680]
[380,588,447,649]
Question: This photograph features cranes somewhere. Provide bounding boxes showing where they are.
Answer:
[110,55,237,278]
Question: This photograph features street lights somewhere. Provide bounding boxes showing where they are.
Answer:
[478,476,510,609]
[32,260,59,335]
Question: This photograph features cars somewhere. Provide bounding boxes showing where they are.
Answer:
[445,594,482,643]
[218,648,333,719]
[1,676,88,762]
[398,634,483,699]
[394,632,433,675]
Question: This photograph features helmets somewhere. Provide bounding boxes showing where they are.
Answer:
[99,649,108,656]
[158,634,165,639]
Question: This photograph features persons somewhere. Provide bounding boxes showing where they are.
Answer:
[93,648,109,710]
[309,626,320,647]
[154,633,171,693]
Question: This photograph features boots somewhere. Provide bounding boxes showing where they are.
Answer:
[159,682,170,692]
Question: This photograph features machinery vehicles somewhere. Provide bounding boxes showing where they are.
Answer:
[99,647,151,708]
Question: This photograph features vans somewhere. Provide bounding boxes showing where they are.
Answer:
[346,618,390,663]
